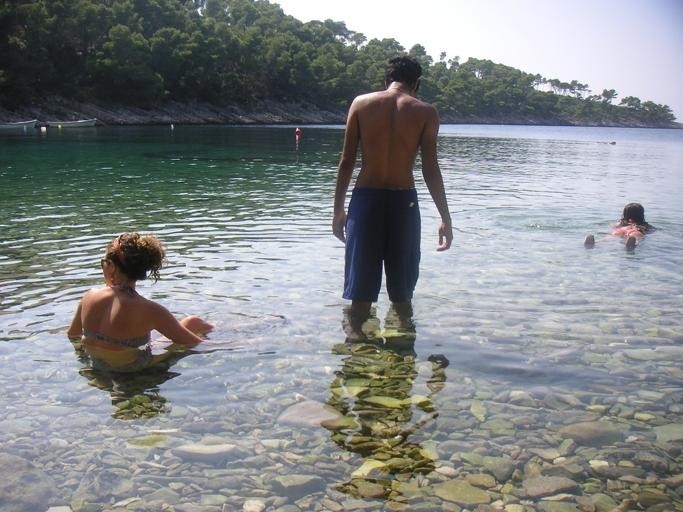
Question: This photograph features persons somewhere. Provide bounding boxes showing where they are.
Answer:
[584,202,658,254]
[331,57,455,321]
[67,233,292,373]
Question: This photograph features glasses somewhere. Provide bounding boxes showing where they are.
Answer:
[101,258,108,268]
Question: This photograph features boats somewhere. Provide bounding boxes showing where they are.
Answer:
[0,118,96,129]
